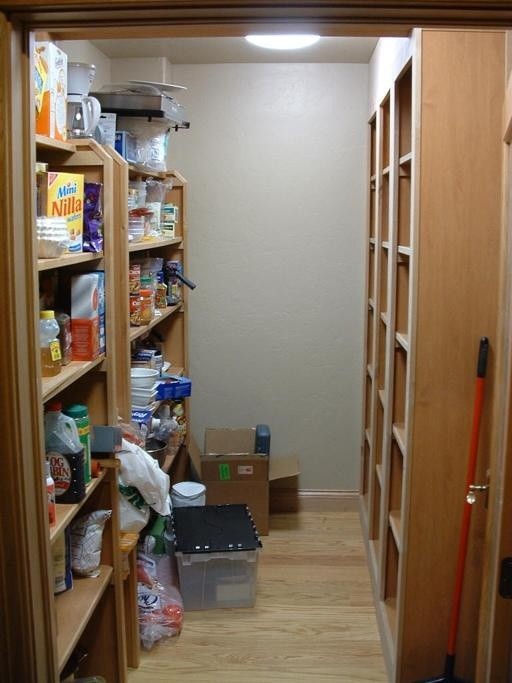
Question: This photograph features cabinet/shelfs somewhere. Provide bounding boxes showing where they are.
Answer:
[33,129,193,683]
[354,24,510,683]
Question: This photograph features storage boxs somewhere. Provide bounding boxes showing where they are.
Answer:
[169,425,301,614]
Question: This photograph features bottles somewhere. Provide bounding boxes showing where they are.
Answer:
[43,461,58,533]
[38,310,62,377]
[61,403,92,488]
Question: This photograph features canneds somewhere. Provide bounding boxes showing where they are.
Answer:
[129,257,182,327]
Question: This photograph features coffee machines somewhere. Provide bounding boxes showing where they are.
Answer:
[65,61,102,139]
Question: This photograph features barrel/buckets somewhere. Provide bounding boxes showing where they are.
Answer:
[43,403,85,504]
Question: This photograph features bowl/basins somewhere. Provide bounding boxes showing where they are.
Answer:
[129,367,160,406]
